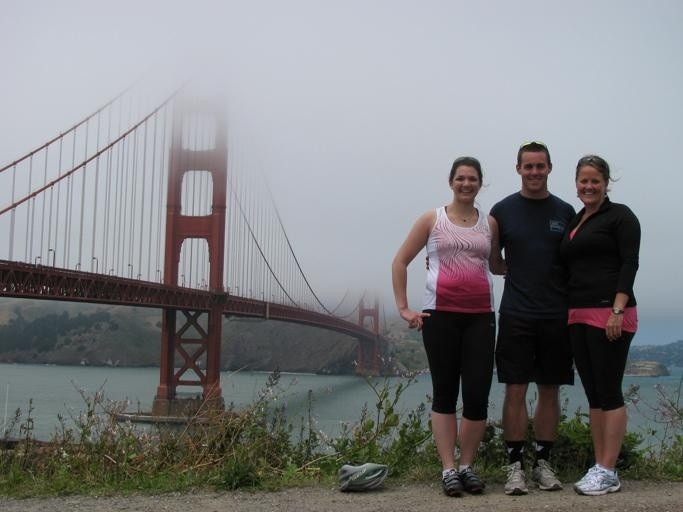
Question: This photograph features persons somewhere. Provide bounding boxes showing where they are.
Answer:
[553,153,642,498]
[390,156,507,497]
[424,140,578,497]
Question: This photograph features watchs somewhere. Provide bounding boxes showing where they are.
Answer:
[610,307,624,315]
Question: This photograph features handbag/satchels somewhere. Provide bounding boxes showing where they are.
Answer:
[338,463,389,492]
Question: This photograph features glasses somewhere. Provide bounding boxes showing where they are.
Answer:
[520,140,546,150]
[454,157,480,165]
[579,157,605,166]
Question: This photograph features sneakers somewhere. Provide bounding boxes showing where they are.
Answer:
[574,466,621,496]
[460,466,485,493]
[531,459,563,491]
[441,467,462,496]
[502,460,529,495]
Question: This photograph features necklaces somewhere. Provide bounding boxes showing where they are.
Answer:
[462,217,467,222]
